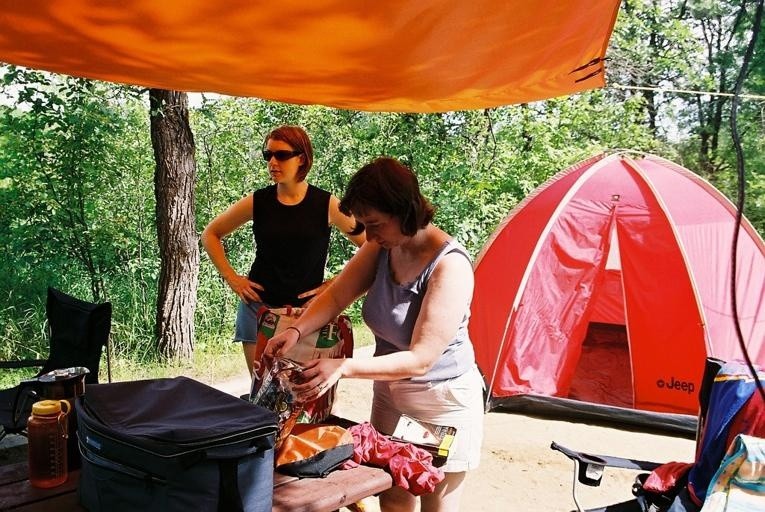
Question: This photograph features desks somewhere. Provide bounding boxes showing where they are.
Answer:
[0,415,405,512]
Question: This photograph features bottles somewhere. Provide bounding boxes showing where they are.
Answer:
[28,399,72,489]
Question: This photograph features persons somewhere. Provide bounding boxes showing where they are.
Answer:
[201,126,366,380]
[391,416,440,447]
[264,157,485,512]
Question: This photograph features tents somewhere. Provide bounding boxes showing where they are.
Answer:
[468,148,765,432]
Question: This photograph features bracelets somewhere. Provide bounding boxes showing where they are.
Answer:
[287,327,300,342]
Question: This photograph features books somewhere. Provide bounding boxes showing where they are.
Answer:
[391,414,457,457]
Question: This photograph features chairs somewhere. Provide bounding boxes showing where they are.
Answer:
[1,286,112,448]
[550,355,749,512]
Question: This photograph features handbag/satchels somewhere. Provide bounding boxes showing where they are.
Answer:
[74,377,280,511]
[249,305,352,427]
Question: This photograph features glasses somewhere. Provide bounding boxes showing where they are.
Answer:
[262,150,302,161]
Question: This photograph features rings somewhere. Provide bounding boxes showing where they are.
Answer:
[317,384,323,391]
